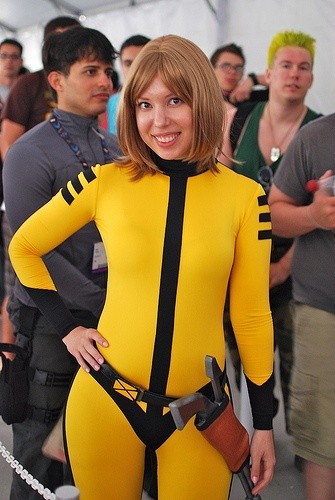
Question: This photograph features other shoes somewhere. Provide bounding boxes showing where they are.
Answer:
[294,454,304,471]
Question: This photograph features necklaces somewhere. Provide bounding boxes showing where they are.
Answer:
[266,106,307,163]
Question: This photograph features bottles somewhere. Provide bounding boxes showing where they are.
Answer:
[306,176,335,196]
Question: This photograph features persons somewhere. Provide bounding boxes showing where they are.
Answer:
[9,36,277,500]
[268,113,335,500]
[209,43,269,154]
[215,31,324,471]
[0,16,83,367]
[0,38,30,115]
[2,27,124,500]
[98,34,151,136]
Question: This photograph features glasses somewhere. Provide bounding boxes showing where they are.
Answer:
[216,63,243,72]
[0,53,21,59]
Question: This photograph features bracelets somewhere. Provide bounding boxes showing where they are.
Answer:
[248,72,258,86]
